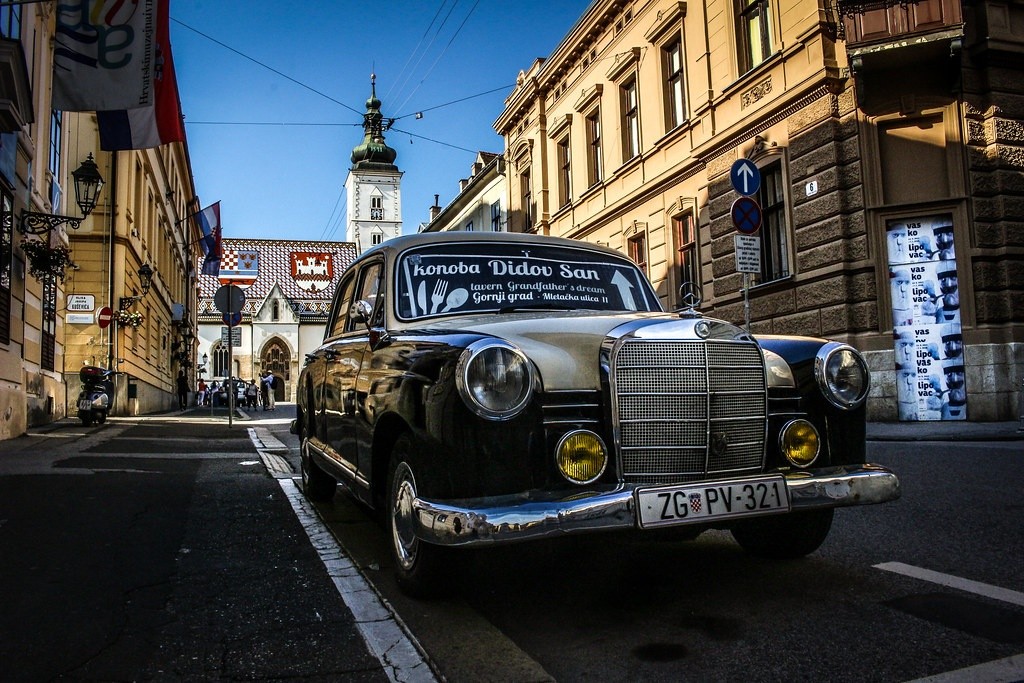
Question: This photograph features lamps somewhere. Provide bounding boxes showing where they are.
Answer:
[15,152,106,237]
[175,310,207,368]
[118,260,155,311]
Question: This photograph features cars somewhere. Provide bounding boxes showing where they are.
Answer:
[291,230,903,601]
[219,379,250,407]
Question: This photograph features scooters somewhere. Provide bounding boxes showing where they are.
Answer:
[76,358,124,428]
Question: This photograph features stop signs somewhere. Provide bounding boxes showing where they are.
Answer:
[95,305,113,329]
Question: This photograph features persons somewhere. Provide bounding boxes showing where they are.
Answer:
[196,379,206,406]
[245,379,258,413]
[175,370,189,412]
[209,379,220,396]
[258,369,276,411]
[260,372,270,410]
[229,375,239,408]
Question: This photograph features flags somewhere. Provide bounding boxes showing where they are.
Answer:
[193,201,224,262]
[95,1,187,153]
[200,236,224,278]
[49,1,161,114]
